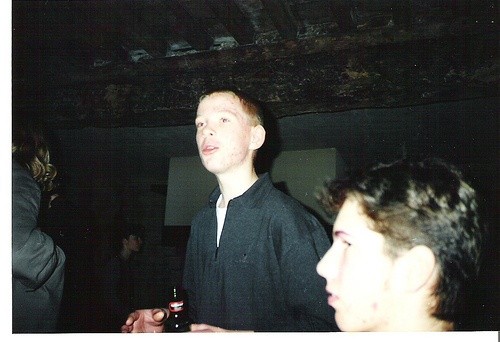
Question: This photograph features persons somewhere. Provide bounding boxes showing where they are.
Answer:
[120,89,341,333]
[100,224,143,333]
[316,158,490,332]
[13,138,67,332]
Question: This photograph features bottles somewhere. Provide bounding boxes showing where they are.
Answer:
[164,288,191,333]
